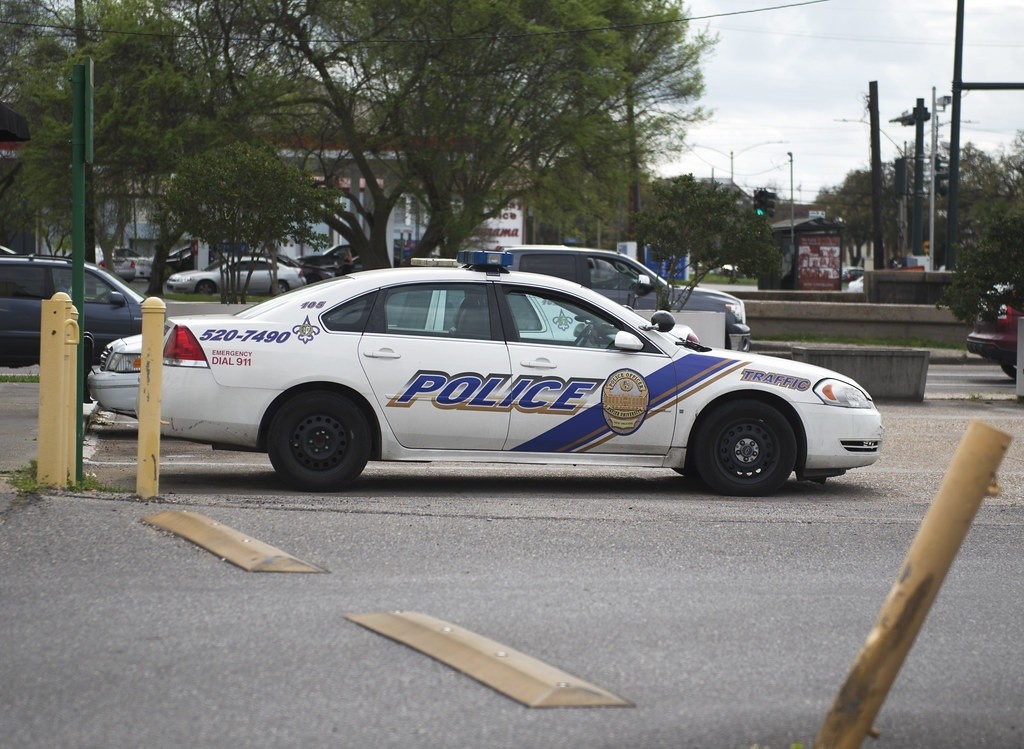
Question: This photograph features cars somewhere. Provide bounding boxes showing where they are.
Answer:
[295,245,402,284]
[112,247,152,278]
[168,247,221,275]
[847,277,864,292]
[92,248,136,282]
[159,247,885,499]
[840,265,865,281]
[966,280,1024,378]
[89,279,700,423]
[167,256,307,297]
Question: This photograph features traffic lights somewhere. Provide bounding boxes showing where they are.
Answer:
[765,192,777,218]
[755,191,766,217]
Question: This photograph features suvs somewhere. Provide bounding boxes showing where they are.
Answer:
[0,254,153,368]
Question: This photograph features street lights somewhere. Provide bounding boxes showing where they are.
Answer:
[692,140,788,183]
[929,86,953,277]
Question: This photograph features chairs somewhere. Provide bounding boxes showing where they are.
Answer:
[456,306,492,340]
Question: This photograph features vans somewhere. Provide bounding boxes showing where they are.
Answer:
[498,245,752,356]
